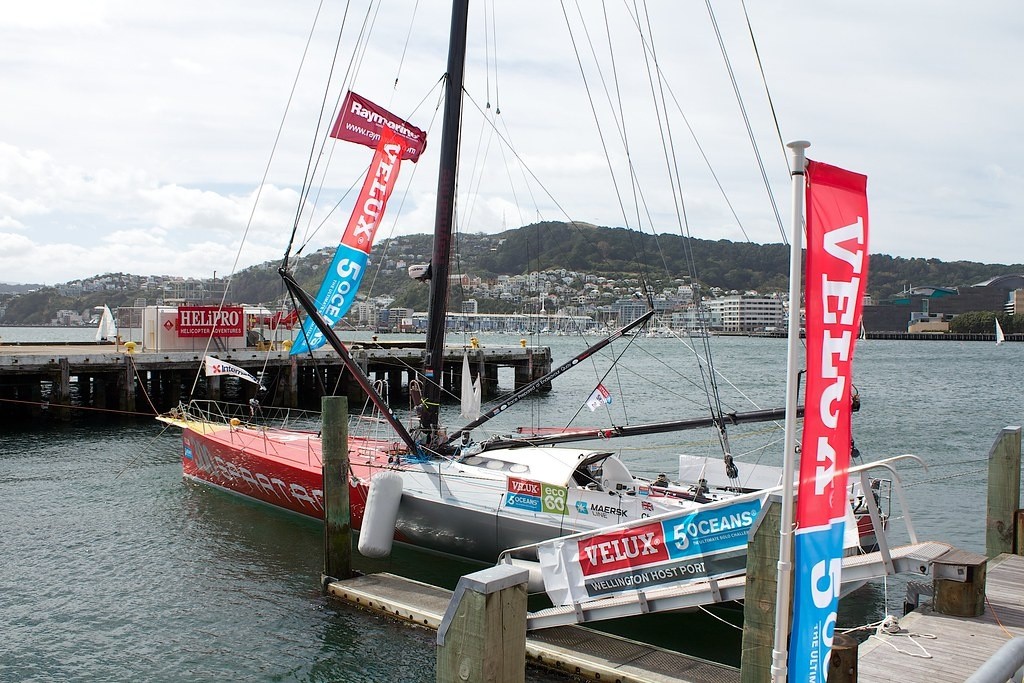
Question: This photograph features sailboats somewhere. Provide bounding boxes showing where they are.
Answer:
[158,0,887,599]
[995,317,1004,346]
